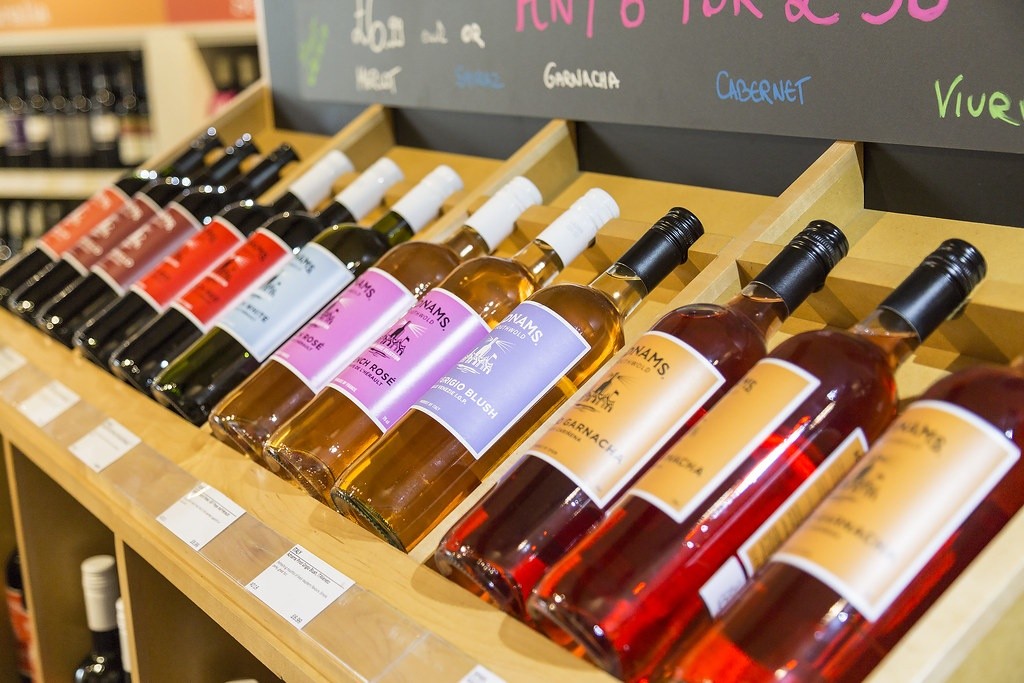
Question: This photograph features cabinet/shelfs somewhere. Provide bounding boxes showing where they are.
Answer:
[0,19,264,199]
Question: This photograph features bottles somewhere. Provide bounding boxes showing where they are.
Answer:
[9,140,263,326]
[110,159,407,401]
[69,150,355,376]
[0,48,257,271]
[4,545,37,683]
[72,555,133,683]
[1,130,231,312]
[35,140,302,348]
[207,175,543,475]
[331,208,706,554]
[262,187,620,511]
[662,349,1024,683]
[525,238,987,682]
[432,220,848,630]
[150,165,463,426]
